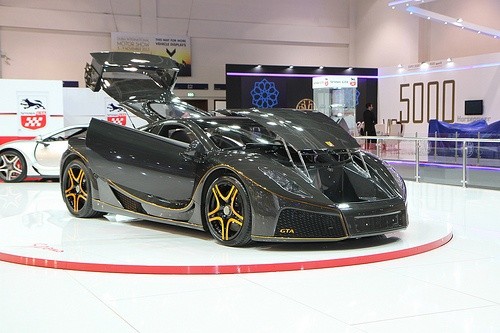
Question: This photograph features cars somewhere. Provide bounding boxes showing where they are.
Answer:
[0,125,93,183]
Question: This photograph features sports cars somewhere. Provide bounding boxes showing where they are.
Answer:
[60,50,409,247]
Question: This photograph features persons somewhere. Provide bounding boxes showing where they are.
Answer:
[362,102,377,143]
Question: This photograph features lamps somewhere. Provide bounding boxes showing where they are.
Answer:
[420,62,430,71]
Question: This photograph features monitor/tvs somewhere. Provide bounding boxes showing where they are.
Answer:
[465,100,483,115]
